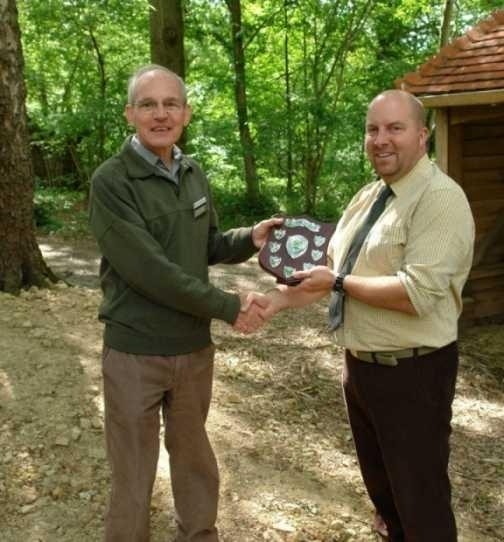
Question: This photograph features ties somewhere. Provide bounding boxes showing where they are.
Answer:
[322,185,392,335]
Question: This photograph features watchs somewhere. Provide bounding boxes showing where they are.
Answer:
[332,273,348,299]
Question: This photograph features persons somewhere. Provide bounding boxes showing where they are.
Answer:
[231,87,478,541]
[84,65,286,542]
